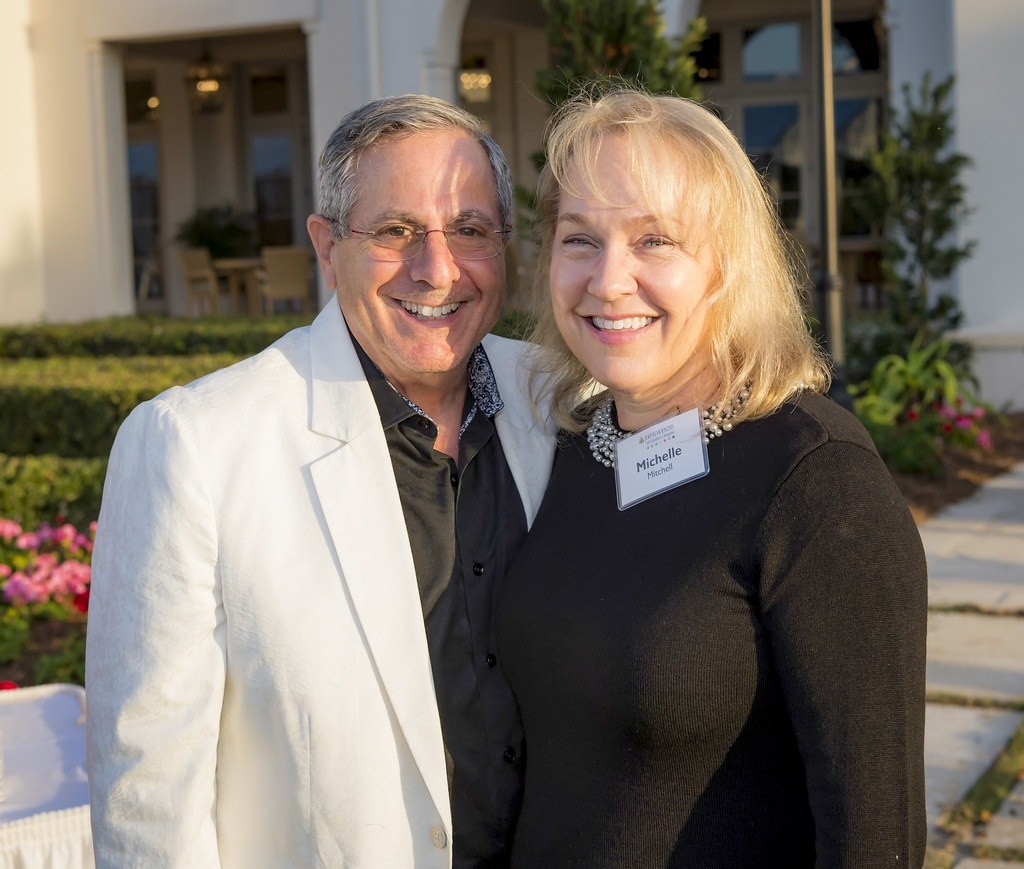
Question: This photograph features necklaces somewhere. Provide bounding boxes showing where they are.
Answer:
[587,380,753,469]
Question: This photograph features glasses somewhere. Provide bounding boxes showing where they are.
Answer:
[329,217,512,258]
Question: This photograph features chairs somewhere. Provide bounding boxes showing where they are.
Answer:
[178,244,315,321]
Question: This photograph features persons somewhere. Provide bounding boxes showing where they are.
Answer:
[494,90,933,869]
[69,85,590,869]
